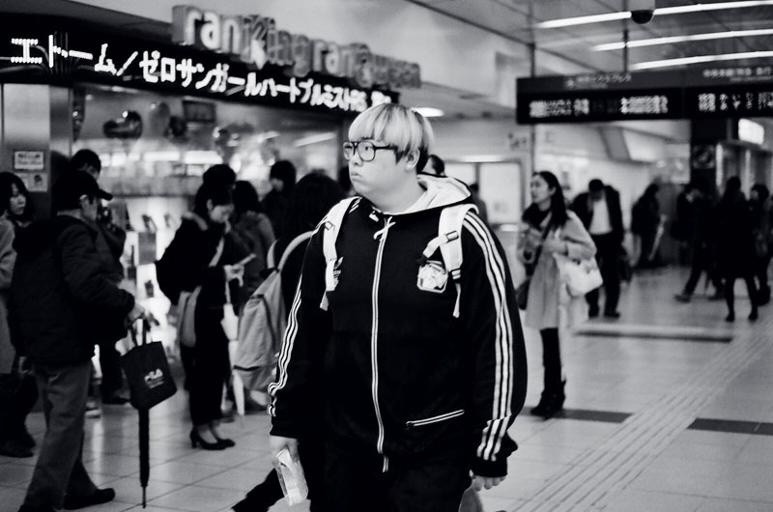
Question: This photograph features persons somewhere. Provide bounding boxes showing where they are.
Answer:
[567,175,773,321]
[265,104,527,511]
[6,174,146,511]
[515,170,602,417]
[0,148,488,461]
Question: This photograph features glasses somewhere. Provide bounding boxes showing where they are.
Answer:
[342,141,398,162]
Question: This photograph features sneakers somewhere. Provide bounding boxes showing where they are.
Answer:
[707,293,718,300]
[674,294,691,303]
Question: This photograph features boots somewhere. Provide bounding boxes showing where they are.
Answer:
[1,375,38,458]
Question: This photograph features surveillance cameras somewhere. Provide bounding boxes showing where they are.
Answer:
[627,0,656,25]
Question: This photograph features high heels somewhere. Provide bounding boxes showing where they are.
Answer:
[531,380,569,419]
[190,426,234,451]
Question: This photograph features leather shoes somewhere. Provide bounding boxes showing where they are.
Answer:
[63,486,117,511]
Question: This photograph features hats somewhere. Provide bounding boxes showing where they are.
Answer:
[51,170,114,202]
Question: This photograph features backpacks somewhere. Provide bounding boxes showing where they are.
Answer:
[232,229,318,391]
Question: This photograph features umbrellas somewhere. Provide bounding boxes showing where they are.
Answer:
[137,317,158,509]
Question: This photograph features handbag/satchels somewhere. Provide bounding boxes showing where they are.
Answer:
[171,291,197,350]
[514,280,532,309]
[554,249,603,300]
[619,247,632,282]
[120,341,177,410]
